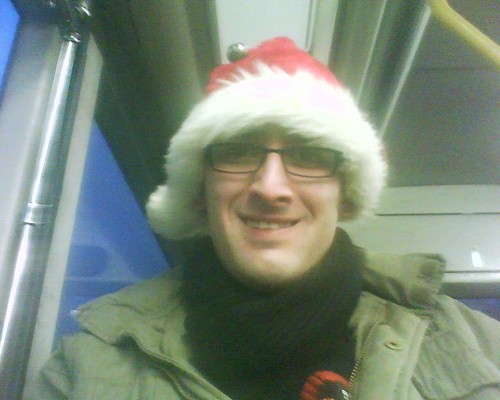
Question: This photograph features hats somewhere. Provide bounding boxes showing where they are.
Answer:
[145,36,388,242]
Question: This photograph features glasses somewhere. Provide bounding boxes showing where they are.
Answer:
[206,144,345,179]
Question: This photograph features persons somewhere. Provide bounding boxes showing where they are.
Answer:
[26,34,500,399]
